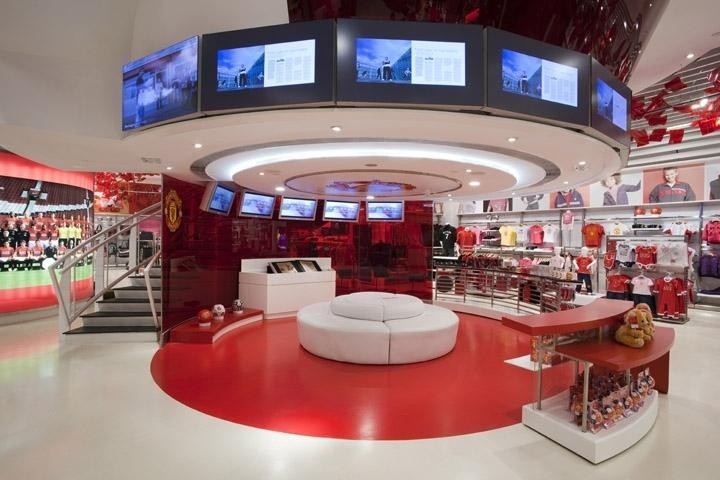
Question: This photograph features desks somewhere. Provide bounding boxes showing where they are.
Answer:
[539,291,598,314]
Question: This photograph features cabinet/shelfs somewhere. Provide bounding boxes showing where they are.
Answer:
[239,257,337,320]
[499,296,675,465]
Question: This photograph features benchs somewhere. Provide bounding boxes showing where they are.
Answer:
[384,290,460,365]
[296,291,390,365]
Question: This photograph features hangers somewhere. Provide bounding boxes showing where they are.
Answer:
[436,212,720,286]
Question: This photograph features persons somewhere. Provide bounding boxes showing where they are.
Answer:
[439,222,457,257]
[361,56,411,82]
[561,210,574,230]
[518,68,530,96]
[486,198,510,212]
[134,64,197,123]
[554,186,584,208]
[549,246,565,269]
[218,63,264,88]
[521,193,545,210]
[572,246,597,296]
[456,226,476,254]
[601,172,641,206]
[1,210,93,271]
[648,167,697,202]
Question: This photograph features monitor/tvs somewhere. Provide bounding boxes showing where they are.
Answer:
[366,200,405,222]
[322,198,361,222]
[591,56,633,150]
[484,26,589,133]
[200,181,237,216]
[239,190,276,219]
[335,18,483,113]
[201,16,334,114]
[279,195,318,222]
[121,35,200,132]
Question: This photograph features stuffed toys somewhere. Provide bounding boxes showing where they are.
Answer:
[635,302,657,334]
[640,309,655,341]
[615,308,649,348]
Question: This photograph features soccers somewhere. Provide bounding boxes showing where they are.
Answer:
[198,309,213,323]
[212,303,225,317]
[232,299,244,310]
